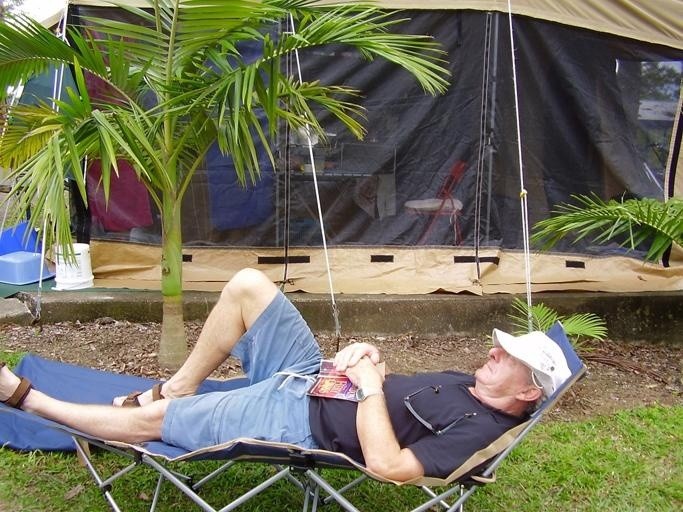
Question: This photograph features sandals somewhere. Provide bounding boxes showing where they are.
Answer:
[0,362,36,412]
[111,383,166,407]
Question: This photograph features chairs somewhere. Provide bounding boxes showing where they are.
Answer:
[399,160,469,245]
[0,315,590,511]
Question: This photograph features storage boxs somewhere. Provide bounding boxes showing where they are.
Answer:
[0,250,55,286]
[0,222,42,256]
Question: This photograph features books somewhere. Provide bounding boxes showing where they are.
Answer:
[306,359,385,402]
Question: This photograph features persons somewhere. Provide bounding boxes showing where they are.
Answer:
[0,266,572,481]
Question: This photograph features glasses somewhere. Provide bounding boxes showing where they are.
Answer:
[403,384,477,435]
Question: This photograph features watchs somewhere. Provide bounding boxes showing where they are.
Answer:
[355,385,381,402]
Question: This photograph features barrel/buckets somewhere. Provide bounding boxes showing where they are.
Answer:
[303,149,326,174]
[52,242,94,290]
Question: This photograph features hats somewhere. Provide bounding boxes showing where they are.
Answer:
[492,327,573,398]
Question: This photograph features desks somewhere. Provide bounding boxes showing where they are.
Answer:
[286,170,373,245]
